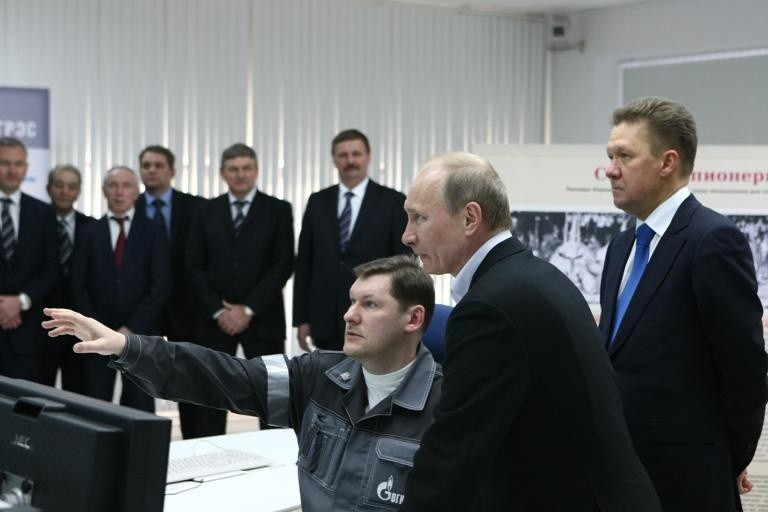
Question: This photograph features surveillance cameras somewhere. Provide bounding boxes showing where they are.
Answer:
[551,17,570,43]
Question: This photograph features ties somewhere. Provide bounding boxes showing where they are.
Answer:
[611,222,655,357]
[149,196,167,242]
[57,217,75,279]
[233,201,246,239]
[113,216,125,266]
[339,192,350,255]
[0,196,16,260]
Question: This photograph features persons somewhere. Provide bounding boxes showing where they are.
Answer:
[594,96,768,511]
[133,145,205,440]
[206,142,292,435]
[401,150,665,511]
[40,252,443,512]
[37,165,96,388]
[0,136,58,383]
[83,165,139,399]
[291,129,418,355]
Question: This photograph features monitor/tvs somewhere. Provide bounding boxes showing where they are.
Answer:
[711,210,768,308]
[0,375,172,512]
[509,207,629,303]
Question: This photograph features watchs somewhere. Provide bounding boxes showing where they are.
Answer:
[244,308,253,314]
